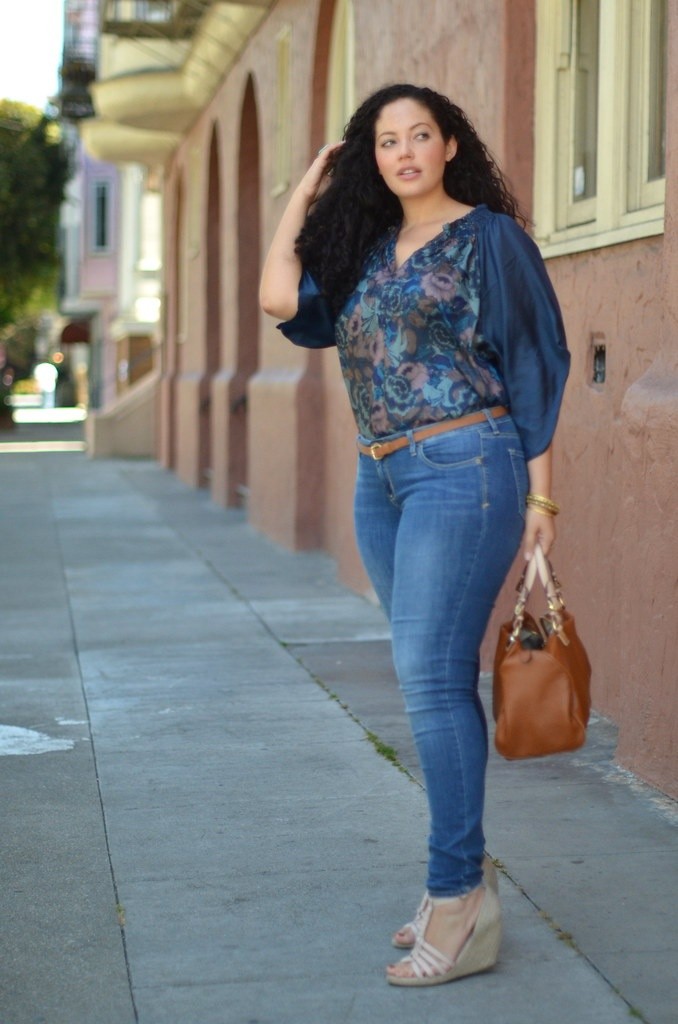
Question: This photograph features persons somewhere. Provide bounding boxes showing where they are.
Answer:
[258,82,575,987]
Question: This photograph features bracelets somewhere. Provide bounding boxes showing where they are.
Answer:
[523,492,561,518]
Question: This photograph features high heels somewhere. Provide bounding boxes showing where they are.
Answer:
[387,849,504,986]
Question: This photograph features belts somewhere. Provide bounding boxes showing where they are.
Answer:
[357,405,509,460]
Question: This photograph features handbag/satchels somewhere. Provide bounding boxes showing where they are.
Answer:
[489,541,591,763]
